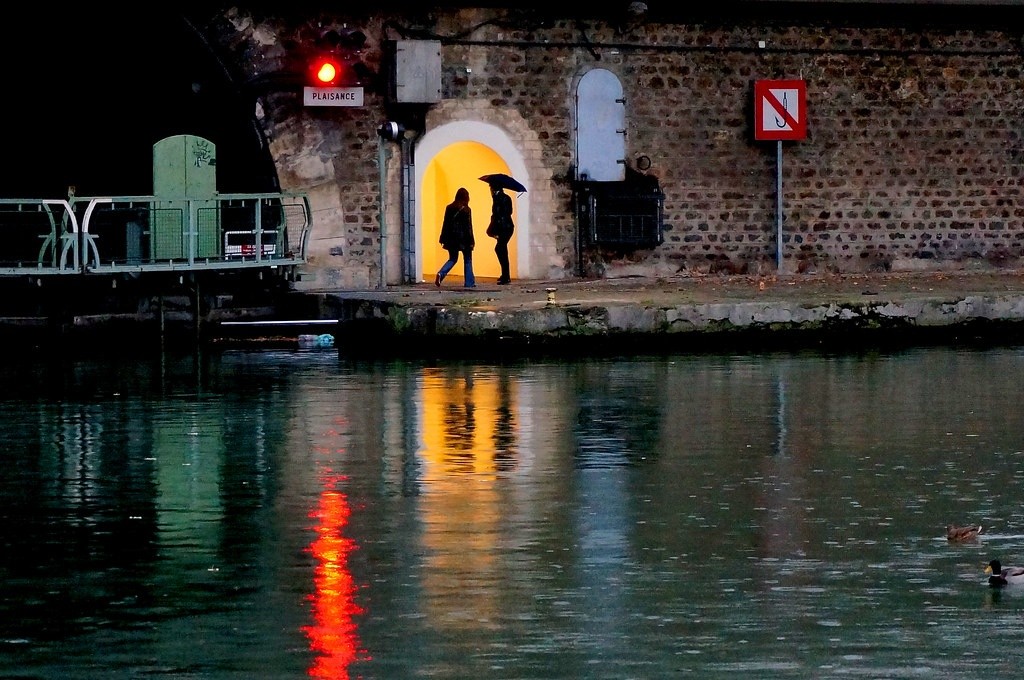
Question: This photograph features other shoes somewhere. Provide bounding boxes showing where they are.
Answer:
[465,283,477,287]
[435,273,441,287]
[497,274,511,285]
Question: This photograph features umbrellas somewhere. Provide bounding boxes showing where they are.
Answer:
[480,172,527,198]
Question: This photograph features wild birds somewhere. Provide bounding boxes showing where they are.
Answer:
[984,559,1024,585]
[943,523,982,542]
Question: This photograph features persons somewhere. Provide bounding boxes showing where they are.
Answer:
[486,182,515,284]
[434,187,478,287]
